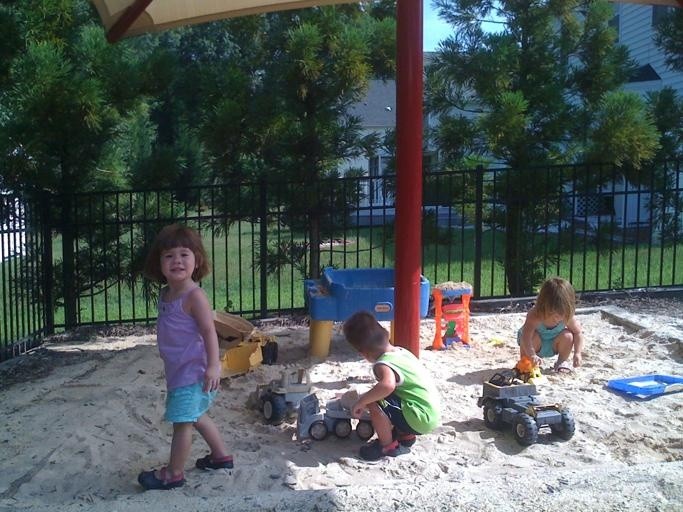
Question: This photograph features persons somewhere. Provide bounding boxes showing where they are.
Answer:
[518,276,583,374]
[138,225,236,489]
[344,310,445,460]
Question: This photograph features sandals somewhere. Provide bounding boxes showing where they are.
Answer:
[137,467,186,489]
[195,454,234,469]
[393,428,417,447]
[360,438,400,460]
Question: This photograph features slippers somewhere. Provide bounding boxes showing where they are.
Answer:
[554,360,574,375]
